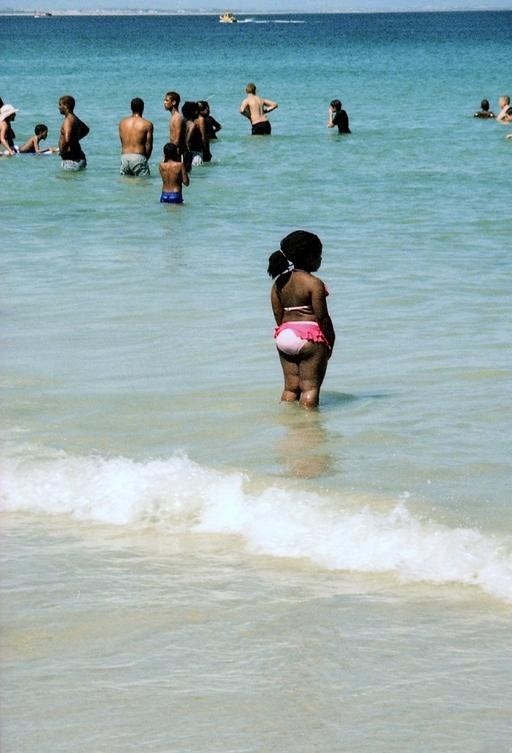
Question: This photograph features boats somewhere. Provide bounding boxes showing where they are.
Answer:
[35,8,53,17]
[216,11,237,23]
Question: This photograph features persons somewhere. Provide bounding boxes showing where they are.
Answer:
[119,97,154,176]
[327,100,351,134]
[159,92,221,203]
[474,96,512,139]
[0,97,59,158]
[58,96,89,171]
[267,231,335,406]
[240,83,278,135]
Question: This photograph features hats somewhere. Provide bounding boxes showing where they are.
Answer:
[0,104,18,121]
